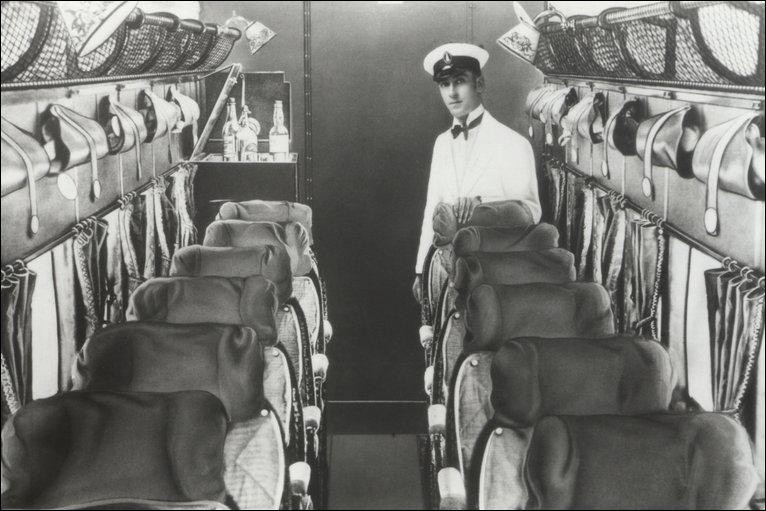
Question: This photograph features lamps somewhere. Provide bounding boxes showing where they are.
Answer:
[225,15,278,56]
[496,8,570,65]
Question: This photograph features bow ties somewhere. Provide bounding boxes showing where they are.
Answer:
[450,112,485,139]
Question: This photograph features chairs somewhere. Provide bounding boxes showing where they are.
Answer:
[418,199,758,510]
[1,200,333,510]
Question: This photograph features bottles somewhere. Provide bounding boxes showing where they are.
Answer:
[222,97,258,161]
[268,100,290,155]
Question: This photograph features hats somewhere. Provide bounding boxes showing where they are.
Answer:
[422,43,490,82]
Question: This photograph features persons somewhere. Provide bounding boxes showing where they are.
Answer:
[411,38,542,307]
